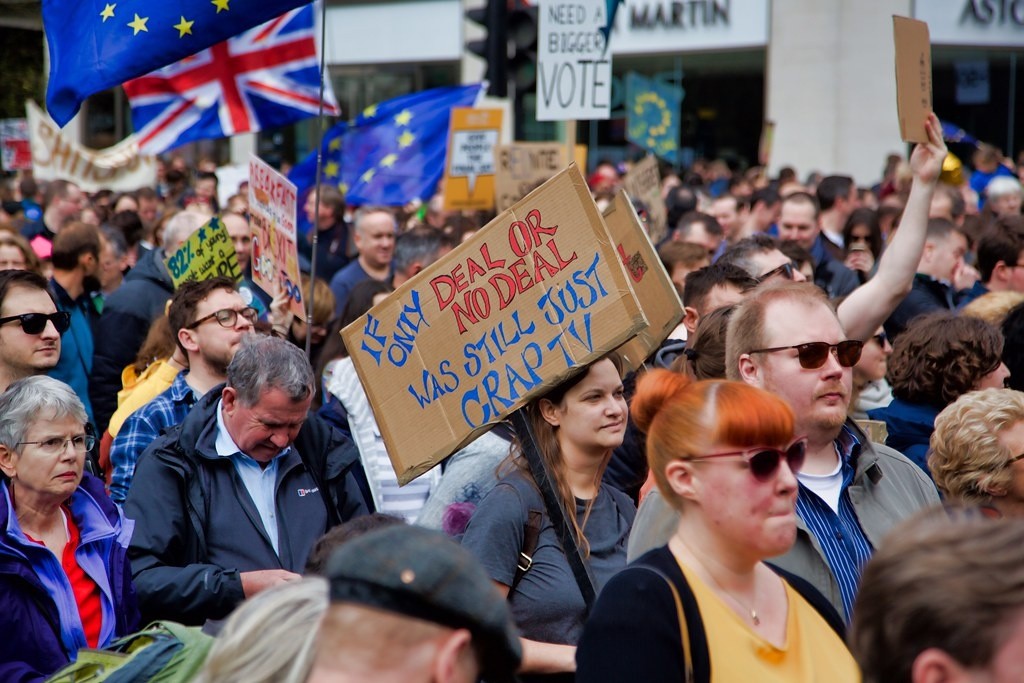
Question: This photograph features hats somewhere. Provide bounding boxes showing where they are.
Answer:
[328,524,524,681]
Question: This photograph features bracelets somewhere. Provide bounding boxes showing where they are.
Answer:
[172,355,190,368]
[271,324,288,341]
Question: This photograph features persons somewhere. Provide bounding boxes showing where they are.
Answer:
[452,349,637,669]
[0,167,590,683]
[575,368,861,682]
[592,113,1023,682]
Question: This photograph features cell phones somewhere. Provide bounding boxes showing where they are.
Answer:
[850,247,864,253]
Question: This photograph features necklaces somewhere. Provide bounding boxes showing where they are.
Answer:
[676,531,762,626]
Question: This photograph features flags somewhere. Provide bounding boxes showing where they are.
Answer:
[287,80,485,234]
[41,1,342,158]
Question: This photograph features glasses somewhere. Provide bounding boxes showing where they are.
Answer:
[753,339,863,368]
[871,331,887,349]
[16,435,95,457]
[851,234,872,243]
[678,435,807,484]
[755,260,798,285]
[187,306,260,328]
[0,311,71,335]
